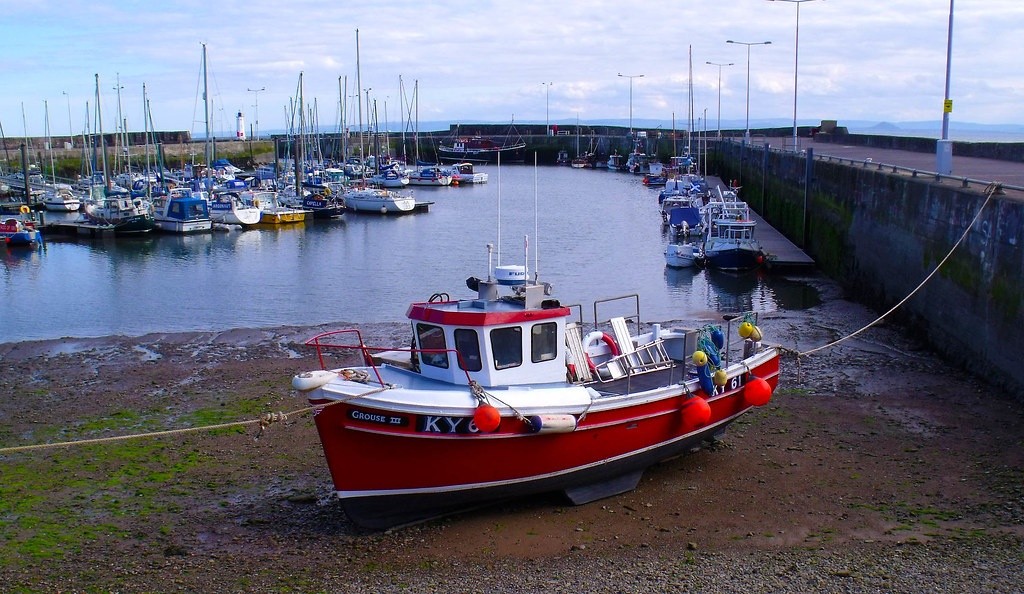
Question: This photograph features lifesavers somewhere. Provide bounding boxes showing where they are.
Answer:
[324,188,331,195]
[581,331,621,377]
[20,205,30,214]
[254,200,260,206]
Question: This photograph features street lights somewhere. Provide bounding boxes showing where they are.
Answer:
[726,40,771,144]
[707,62,735,139]
[247,88,264,140]
[64,92,74,146]
[542,82,552,135]
[617,73,645,132]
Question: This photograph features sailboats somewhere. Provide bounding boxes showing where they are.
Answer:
[0,25,452,235]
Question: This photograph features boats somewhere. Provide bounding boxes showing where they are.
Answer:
[439,113,526,165]
[446,162,489,184]
[0,220,40,251]
[664,241,695,269]
[293,234,779,525]
[701,200,762,272]
[558,131,712,240]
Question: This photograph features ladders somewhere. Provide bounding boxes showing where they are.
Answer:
[595,337,676,384]
